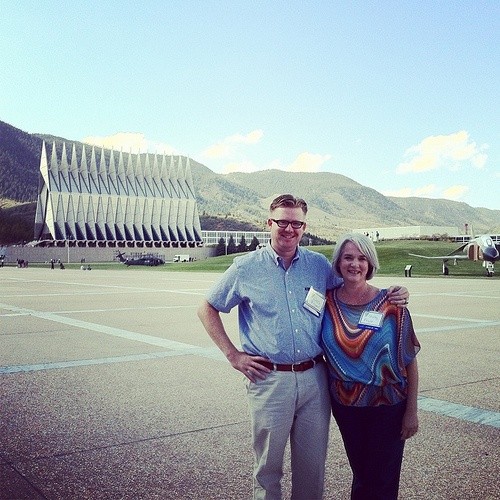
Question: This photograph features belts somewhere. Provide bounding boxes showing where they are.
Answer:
[253,354,323,373]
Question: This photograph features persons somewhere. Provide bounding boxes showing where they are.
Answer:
[197,194,409,500]
[0,254,4,268]
[364,230,380,243]
[50,256,65,269]
[80,257,93,272]
[319,233,421,500]
[183,256,198,263]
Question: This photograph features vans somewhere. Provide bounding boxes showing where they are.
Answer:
[173,255,190,262]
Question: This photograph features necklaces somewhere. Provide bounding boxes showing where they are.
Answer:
[340,285,369,306]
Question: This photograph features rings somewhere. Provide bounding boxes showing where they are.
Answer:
[406,299,408,305]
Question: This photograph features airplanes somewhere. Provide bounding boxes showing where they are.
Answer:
[404,224,500,277]
[115,248,165,267]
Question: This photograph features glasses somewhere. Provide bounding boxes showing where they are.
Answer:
[271,219,306,229]
